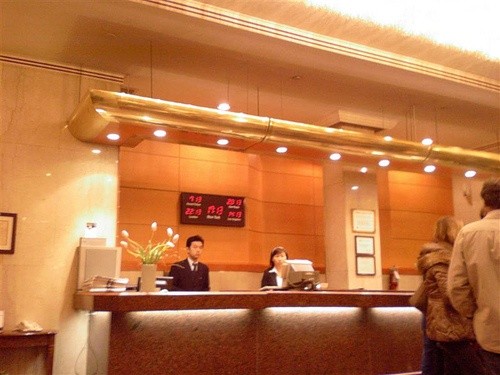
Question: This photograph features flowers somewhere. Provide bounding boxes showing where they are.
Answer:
[119,222,179,264]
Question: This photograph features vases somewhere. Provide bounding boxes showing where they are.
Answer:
[140,262,158,292]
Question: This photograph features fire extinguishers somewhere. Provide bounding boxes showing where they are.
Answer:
[389,265,399,290]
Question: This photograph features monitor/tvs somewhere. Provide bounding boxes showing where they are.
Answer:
[282,260,314,287]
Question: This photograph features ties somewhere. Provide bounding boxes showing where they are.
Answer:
[192,263,198,271]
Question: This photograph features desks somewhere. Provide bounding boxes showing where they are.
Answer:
[0,331,56,375]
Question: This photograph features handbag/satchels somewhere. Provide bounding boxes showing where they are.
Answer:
[409,280,428,314]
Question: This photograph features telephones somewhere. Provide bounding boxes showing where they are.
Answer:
[17,322,43,331]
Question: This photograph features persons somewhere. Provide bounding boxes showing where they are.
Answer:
[416,177,500,375]
[168,236,210,291]
[261,246,289,291]
